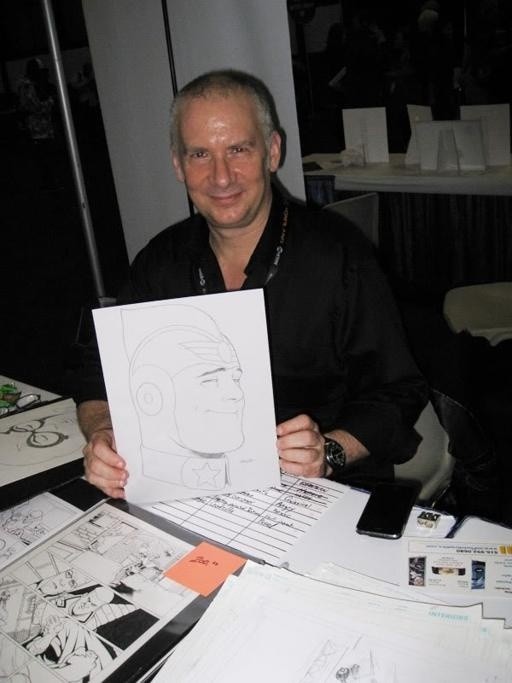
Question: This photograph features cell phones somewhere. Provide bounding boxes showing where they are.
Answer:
[355,477,423,538]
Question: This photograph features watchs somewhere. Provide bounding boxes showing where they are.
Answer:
[321,436,346,477]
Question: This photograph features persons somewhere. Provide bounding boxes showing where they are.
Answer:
[72,66,433,511]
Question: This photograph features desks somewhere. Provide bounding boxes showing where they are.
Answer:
[0,376,511,681]
